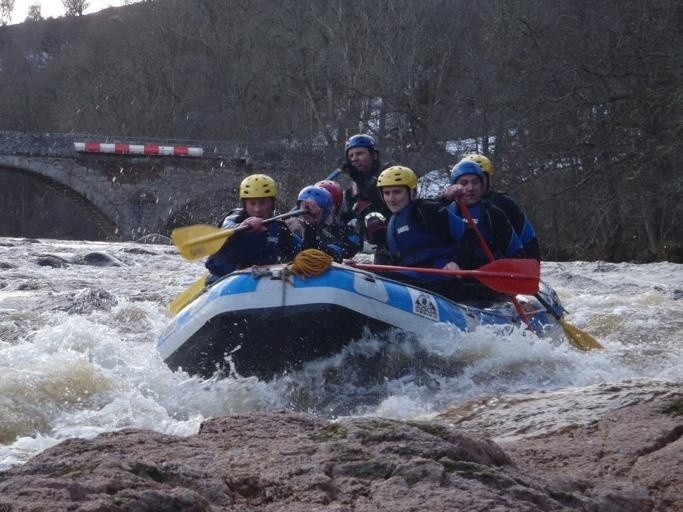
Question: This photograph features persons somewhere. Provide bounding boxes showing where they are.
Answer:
[203,133,540,310]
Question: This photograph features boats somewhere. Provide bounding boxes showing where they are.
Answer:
[154,260,564,390]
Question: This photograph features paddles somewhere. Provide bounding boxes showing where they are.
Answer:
[532,290,602,350]
[352,257,539,299]
[167,166,340,314]
[171,207,311,258]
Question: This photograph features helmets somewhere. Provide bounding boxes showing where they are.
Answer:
[376,165,418,189]
[463,154,495,176]
[345,134,375,153]
[239,174,278,200]
[315,180,343,210]
[450,160,484,184]
[297,185,333,217]
